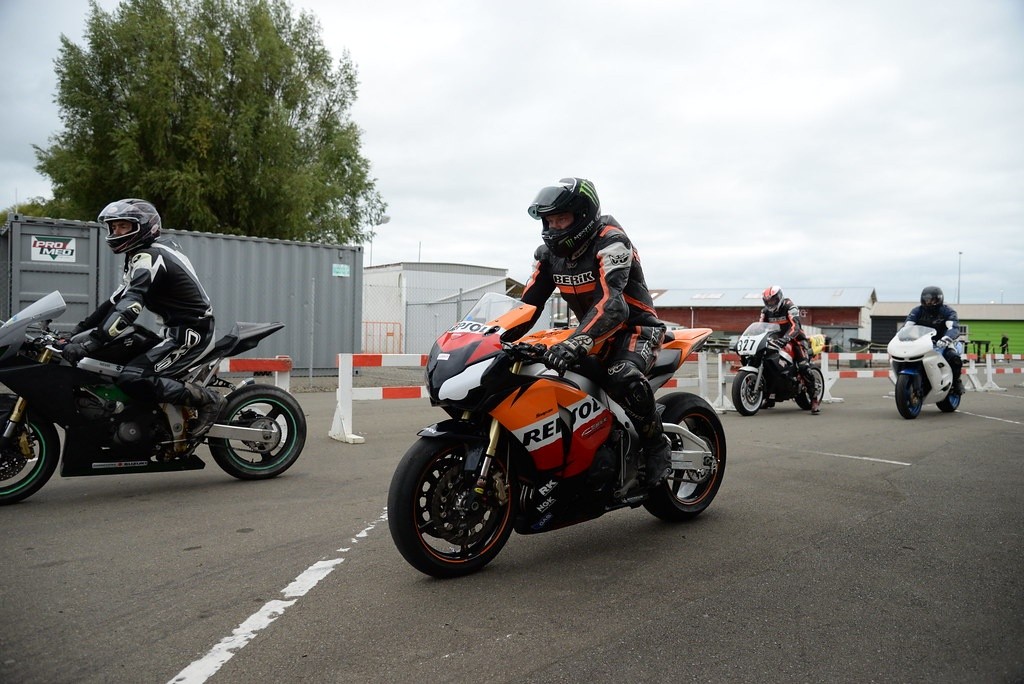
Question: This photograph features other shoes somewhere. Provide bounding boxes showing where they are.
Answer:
[953,379,965,395]
[635,433,672,492]
[760,399,767,409]
[811,402,821,414]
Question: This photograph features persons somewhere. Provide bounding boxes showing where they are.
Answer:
[899,286,966,395]
[1000,333,1008,354]
[502,179,671,485]
[62,199,227,435]
[754,286,821,415]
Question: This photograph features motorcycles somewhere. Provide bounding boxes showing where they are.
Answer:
[0,290,308,505]
[887,325,963,419]
[387,292,727,580]
[728,322,825,416]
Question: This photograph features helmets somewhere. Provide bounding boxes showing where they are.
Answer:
[533,178,601,262]
[921,286,943,310]
[104,199,161,254]
[763,286,784,313]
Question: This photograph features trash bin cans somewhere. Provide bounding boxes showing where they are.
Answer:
[849,338,869,368]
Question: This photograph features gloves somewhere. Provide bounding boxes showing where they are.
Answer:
[773,338,787,348]
[62,325,83,341]
[936,340,948,349]
[489,326,519,343]
[61,340,99,368]
[543,335,594,378]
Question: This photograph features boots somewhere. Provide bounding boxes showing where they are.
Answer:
[181,382,228,438]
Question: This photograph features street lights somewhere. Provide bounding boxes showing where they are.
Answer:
[958,252,963,304]
